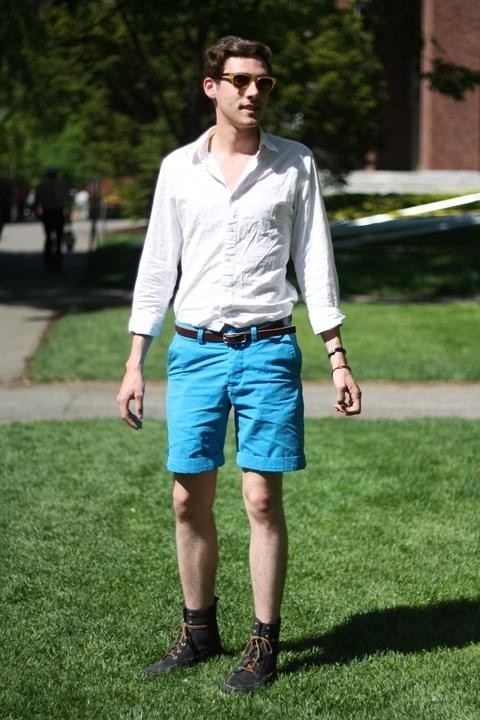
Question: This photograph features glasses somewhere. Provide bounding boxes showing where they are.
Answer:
[222,70,280,94]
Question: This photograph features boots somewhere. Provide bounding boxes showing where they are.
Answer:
[141,594,224,676]
[222,616,290,694]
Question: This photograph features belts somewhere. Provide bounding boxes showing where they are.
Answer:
[174,313,298,347]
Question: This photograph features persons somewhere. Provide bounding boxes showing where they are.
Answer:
[30,163,66,268]
[115,34,363,697]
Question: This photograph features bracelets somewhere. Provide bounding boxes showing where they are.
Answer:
[330,364,352,376]
[327,347,347,359]
